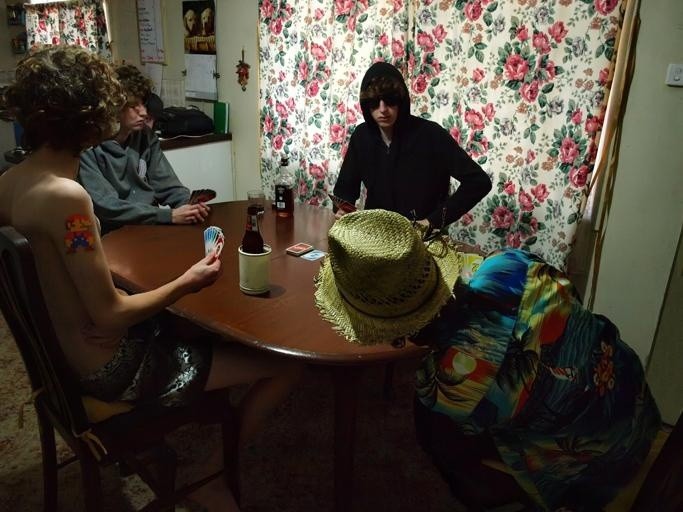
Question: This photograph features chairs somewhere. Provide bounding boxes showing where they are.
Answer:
[1,227,238,512]
[600,361,683,512]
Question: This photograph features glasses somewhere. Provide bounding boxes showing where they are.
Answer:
[363,93,400,108]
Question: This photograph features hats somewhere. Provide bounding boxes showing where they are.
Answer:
[316,208,459,341]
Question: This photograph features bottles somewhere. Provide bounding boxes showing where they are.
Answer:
[273,155,295,218]
[242,206,263,253]
[8,4,17,23]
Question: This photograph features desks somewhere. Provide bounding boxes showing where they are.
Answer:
[101,198,488,512]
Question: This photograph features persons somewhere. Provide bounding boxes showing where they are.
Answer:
[330,61,493,234]
[314,209,663,512]
[0,42,291,484]
[78,62,212,237]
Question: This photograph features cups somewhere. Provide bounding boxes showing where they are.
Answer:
[247,190,265,215]
[238,244,272,295]
